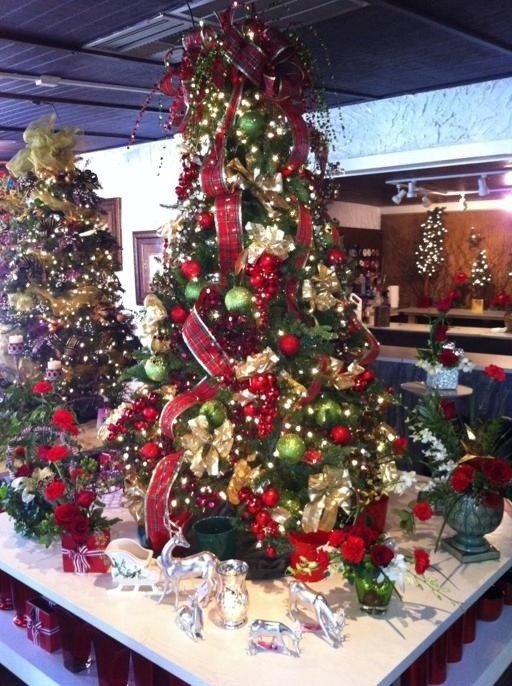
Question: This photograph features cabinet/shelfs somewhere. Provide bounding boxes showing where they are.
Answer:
[0,468,512,685]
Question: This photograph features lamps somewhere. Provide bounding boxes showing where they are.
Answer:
[385,168,491,211]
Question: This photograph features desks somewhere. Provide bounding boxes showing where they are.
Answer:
[367,307,512,496]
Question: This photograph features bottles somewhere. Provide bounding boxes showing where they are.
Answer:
[212,557,251,632]
